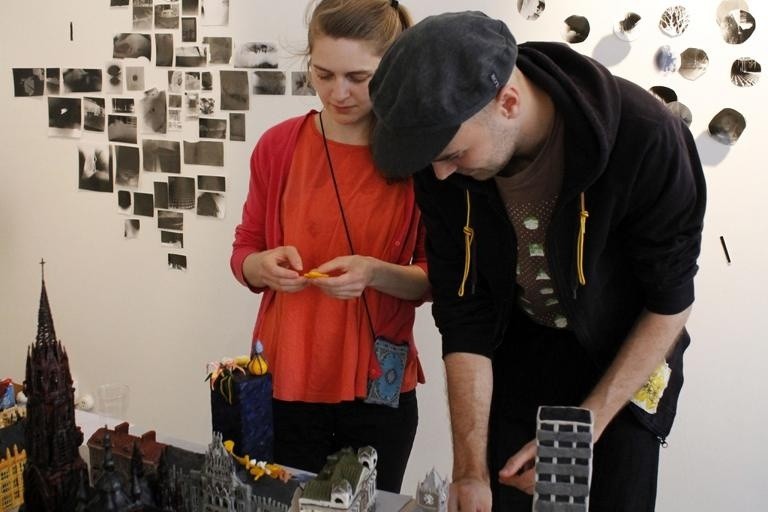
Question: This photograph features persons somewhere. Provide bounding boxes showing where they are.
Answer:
[230,0,433,495]
[369,10,706,512]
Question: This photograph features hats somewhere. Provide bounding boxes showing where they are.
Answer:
[369,10,517,180]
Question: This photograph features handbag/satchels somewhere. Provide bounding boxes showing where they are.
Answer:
[363,336,409,409]
